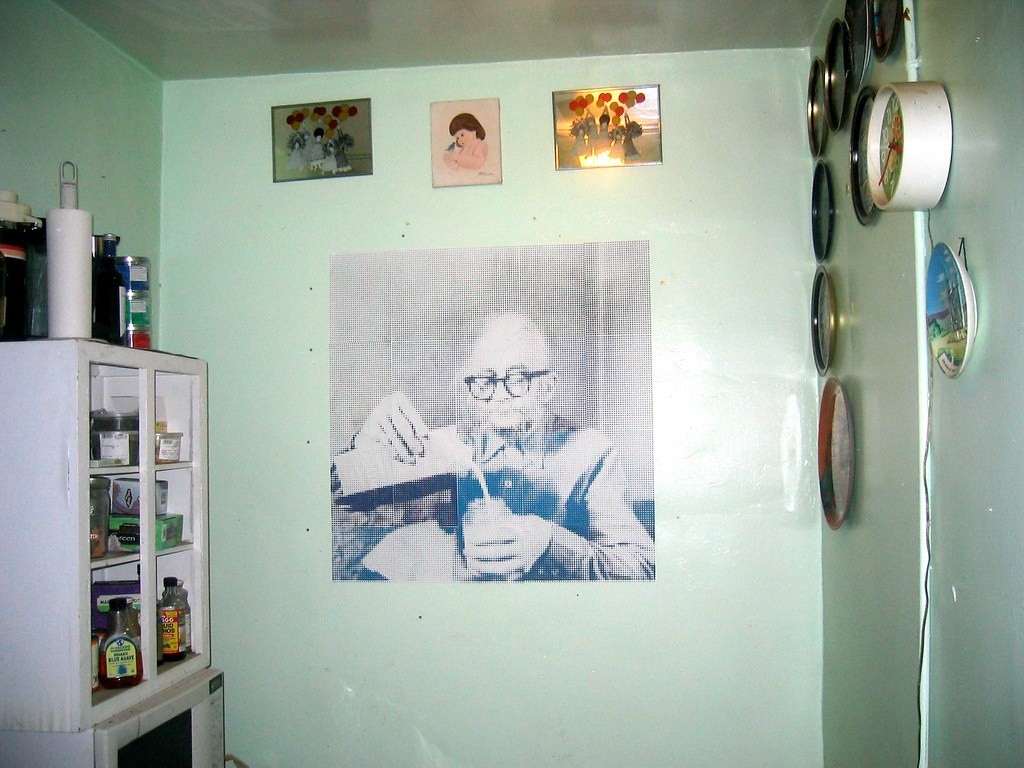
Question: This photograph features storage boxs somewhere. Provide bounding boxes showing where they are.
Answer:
[92,580,141,629]
[112,478,168,515]
[107,514,183,550]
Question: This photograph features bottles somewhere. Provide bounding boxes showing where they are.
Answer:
[462,496,514,578]
[0,189,42,342]
[99,597,143,689]
[92,233,126,346]
[160,576,186,662]
[89,477,111,558]
[45,160,92,339]
[124,597,141,644]
[162,580,191,654]
[91,628,100,692]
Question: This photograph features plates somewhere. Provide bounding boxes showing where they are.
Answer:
[926,241,978,379]
[805,0,903,532]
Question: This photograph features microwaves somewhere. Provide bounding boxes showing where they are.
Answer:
[0,668,225,768]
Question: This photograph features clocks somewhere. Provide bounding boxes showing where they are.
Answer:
[867,83,951,211]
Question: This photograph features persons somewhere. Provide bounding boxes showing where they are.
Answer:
[330,315,655,580]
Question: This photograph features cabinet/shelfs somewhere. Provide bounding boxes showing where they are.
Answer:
[0,339,209,734]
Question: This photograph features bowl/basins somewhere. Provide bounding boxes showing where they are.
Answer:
[90,407,184,467]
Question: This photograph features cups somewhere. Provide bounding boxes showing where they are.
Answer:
[116,255,152,350]
